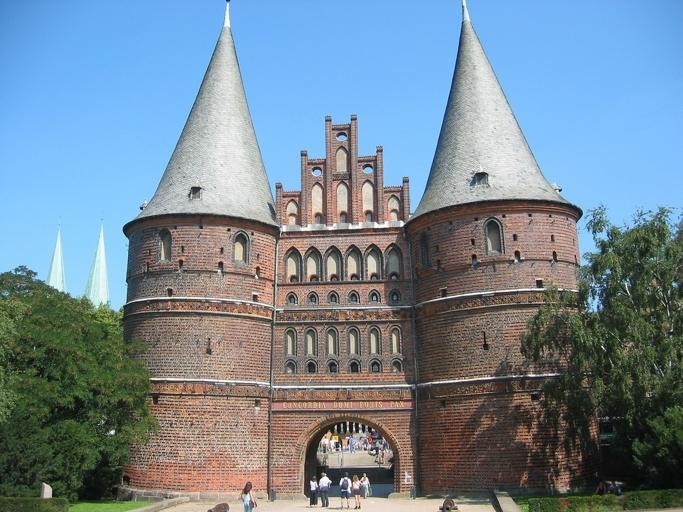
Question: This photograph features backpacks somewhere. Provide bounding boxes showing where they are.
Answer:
[342,477,348,489]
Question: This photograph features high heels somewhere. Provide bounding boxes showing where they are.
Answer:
[355,506,360,509]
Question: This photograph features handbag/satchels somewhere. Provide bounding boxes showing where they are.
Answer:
[359,485,363,490]
[249,500,257,507]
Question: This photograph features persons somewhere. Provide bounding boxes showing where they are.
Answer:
[319,431,387,464]
[310,472,332,507]
[339,472,370,509]
[241,482,257,512]
